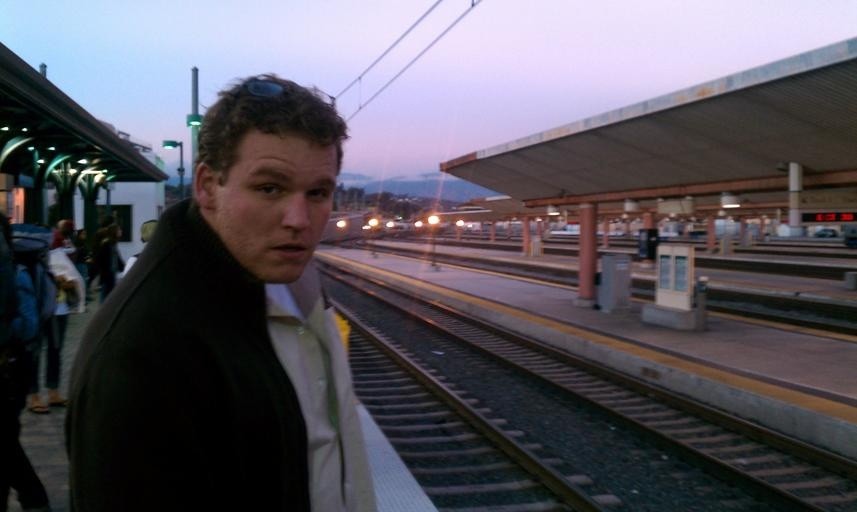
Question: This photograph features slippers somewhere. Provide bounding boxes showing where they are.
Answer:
[28,399,68,414]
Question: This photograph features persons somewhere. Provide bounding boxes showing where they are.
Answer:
[1,206,127,509]
[61,73,347,510]
[123,219,158,275]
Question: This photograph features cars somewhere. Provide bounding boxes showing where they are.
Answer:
[815,228,837,238]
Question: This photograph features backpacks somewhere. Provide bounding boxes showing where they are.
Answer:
[6,224,59,327]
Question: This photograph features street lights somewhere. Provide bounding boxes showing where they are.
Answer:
[429,217,439,266]
[187,114,203,127]
[162,140,185,201]
[369,219,377,256]
